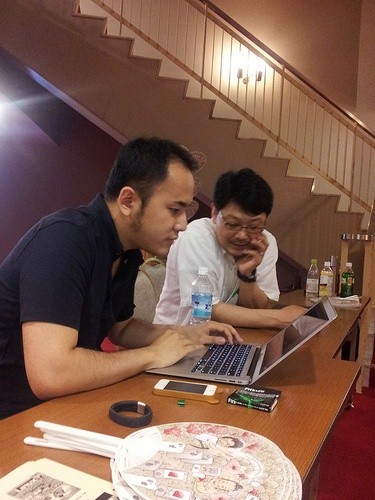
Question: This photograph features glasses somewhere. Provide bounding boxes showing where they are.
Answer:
[219,208,266,234]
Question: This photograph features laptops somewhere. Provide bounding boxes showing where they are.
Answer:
[145,295,338,386]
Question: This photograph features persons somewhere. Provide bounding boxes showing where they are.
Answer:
[0,136,245,421]
[152,168,309,345]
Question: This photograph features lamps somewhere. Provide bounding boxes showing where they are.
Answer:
[236,65,263,84]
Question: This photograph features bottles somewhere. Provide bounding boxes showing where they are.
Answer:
[305,259,319,305]
[190,266,213,325]
[319,262,333,297]
[340,263,355,298]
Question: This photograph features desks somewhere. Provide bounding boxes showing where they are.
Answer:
[210,289,371,410]
[0,358,362,500]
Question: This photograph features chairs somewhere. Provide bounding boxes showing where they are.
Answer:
[134,257,166,322]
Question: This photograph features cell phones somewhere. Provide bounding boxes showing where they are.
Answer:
[152,380,218,402]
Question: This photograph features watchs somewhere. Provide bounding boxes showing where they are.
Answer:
[236,268,258,283]
